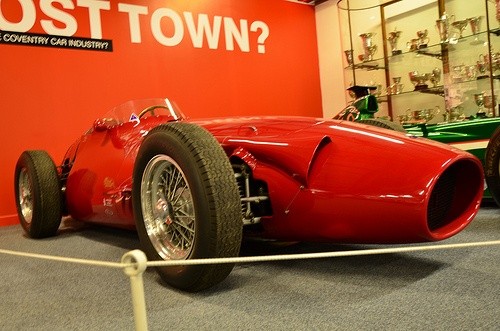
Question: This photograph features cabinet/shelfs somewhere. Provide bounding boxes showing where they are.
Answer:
[336,0,500,124]
